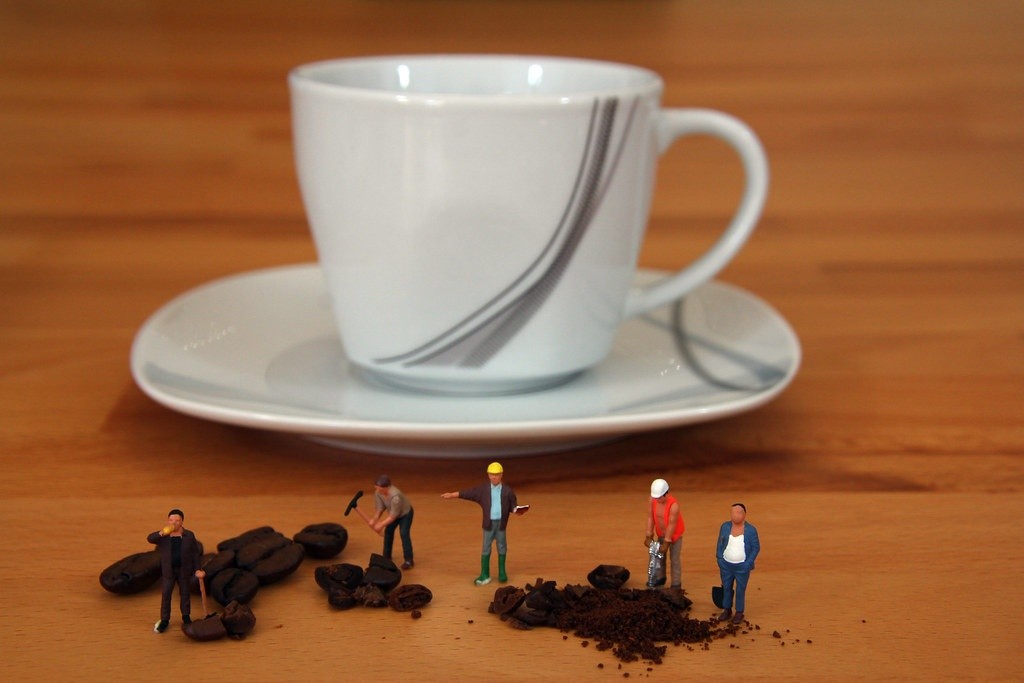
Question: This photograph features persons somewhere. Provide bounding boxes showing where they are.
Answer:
[368,475,415,570]
[440,461,526,586]
[146,507,204,637]
[716,503,761,624]
[644,478,686,591]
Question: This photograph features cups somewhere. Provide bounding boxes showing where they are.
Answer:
[290,55,768,397]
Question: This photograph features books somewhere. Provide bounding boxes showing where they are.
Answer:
[513,504,531,515]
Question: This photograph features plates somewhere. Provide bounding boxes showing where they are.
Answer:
[130,263,802,454]
